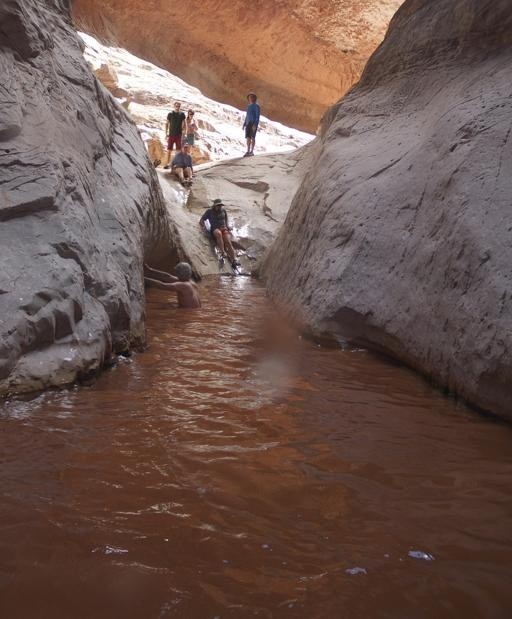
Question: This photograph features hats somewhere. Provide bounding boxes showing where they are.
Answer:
[210,198,226,207]
[246,92,257,101]
[172,261,191,273]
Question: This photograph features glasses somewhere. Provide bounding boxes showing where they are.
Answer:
[188,113,194,115]
[175,105,180,108]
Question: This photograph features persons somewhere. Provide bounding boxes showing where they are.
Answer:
[182,107,202,154]
[169,141,196,185]
[142,260,202,308]
[198,198,242,268]
[163,101,187,170]
[241,92,261,157]
